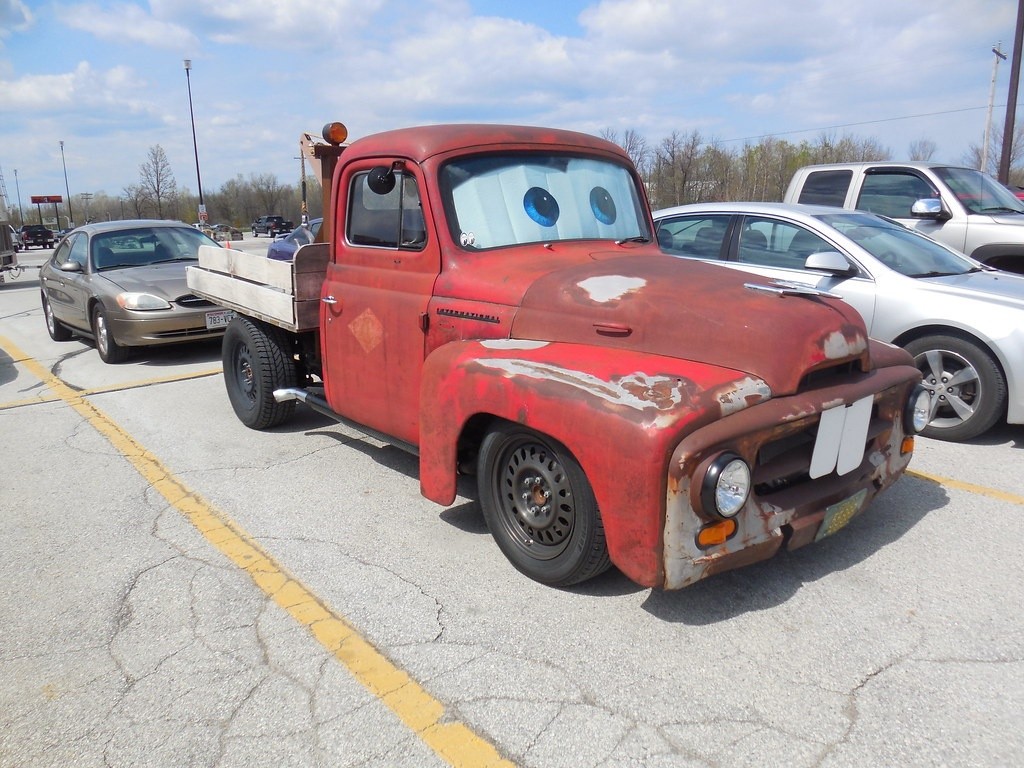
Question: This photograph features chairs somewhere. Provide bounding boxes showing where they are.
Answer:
[788,231,819,258]
[154,244,168,259]
[99,247,114,266]
[742,230,768,250]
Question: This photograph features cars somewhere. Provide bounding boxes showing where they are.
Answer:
[644,203,1023,444]
[39,219,242,364]
[7,225,22,254]
[208,223,245,242]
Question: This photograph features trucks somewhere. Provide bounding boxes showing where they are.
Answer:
[188,123,931,601]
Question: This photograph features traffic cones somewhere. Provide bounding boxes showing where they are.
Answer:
[225,240,231,249]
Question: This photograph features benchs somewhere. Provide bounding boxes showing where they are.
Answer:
[657,227,719,254]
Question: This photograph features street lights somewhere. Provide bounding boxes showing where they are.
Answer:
[13,169,24,226]
[184,57,209,224]
[60,140,75,227]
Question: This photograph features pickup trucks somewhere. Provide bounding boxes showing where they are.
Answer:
[18,224,56,250]
[707,162,1023,293]
[251,215,295,238]
